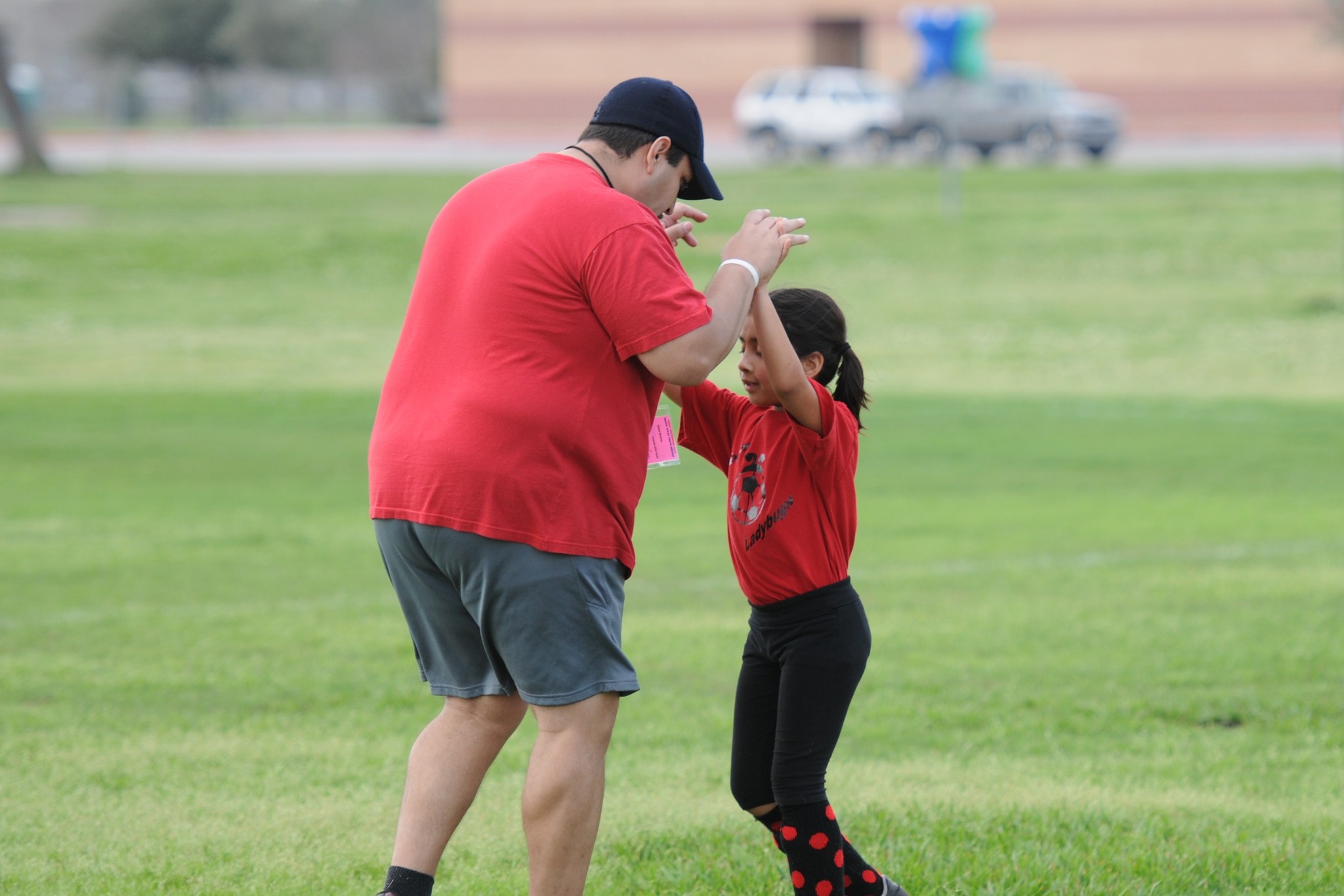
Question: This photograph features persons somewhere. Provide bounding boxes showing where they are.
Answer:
[662,286,913,895]
[369,77,811,896]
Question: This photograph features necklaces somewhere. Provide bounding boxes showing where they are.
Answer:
[564,145,613,189]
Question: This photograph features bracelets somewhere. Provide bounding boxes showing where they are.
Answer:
[716,256,759,290]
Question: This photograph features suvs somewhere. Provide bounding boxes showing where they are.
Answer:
[736,64,1123,161]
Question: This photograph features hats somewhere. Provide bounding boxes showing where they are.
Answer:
[590,76,724,201]
